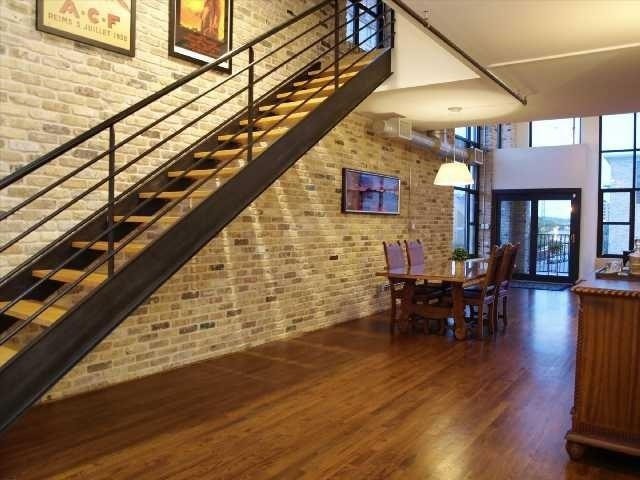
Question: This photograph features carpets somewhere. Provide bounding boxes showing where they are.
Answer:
[500,280,570,292]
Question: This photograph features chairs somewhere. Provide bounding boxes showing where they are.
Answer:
[385,239,520,340]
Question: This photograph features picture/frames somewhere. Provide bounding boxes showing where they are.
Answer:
[33,1,235,74]
[341,167,401,216]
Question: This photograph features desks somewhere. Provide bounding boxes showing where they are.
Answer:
[564,276,640,468]
[375,260,488,344]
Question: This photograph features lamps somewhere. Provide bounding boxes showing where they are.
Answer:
[432,129,473,188]
